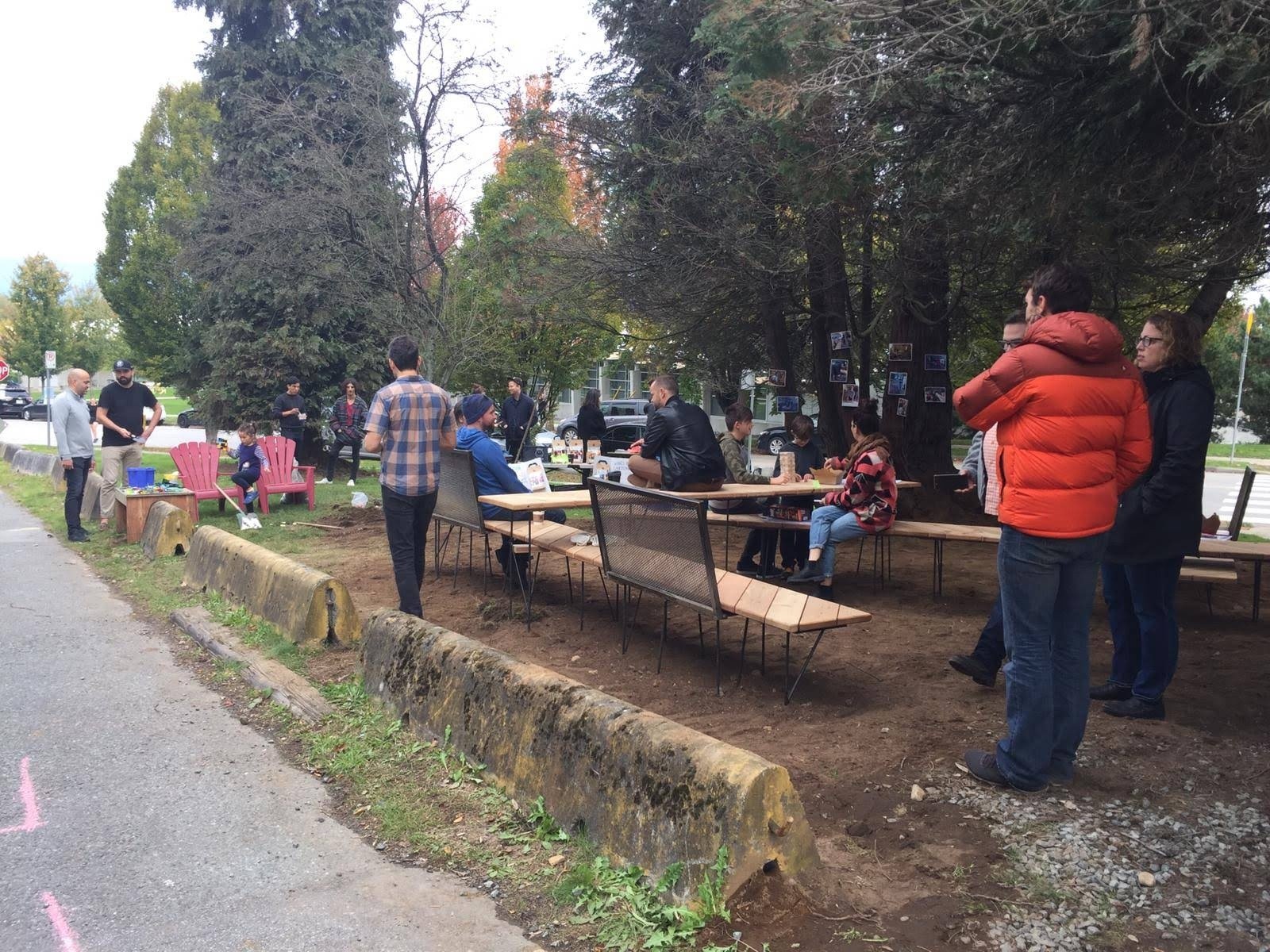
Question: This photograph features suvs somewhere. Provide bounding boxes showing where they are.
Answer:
[557,398,650,447]
[0,383,32,419]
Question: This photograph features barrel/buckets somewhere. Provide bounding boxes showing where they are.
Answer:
[127,467,156,488]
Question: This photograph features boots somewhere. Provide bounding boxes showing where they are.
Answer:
[787,557,826,583]
[812,584,834,601]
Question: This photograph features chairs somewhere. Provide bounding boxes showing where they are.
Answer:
[255,435,316,514]
[171,441,246,515]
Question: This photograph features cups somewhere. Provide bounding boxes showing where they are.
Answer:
[532,511,544,526]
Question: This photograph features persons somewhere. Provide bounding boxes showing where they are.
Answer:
[272,376,308,471]
[926,393,938,401]
[94,359,161,531]
[362,330,456,621]
[456,377,898,600]
[225,423,272,515]
[949,309,1029,689]
[311,379,369,487]
[627,377,729,513]
[947,258,1153,791]
[88,397,99,443]
[50,368,95,543]
[1085,308,1217,718]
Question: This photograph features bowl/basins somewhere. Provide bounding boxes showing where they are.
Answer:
[298,414,307,420]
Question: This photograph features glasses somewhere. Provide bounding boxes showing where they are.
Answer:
[998,339,1023,349]
[1135,335,1165,347]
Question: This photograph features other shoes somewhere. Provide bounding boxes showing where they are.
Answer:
[99,519,109,531]
[496,543,529,586]
[780,564,794,579]
[949,652,1000,687]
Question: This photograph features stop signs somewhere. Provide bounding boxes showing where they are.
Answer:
[0,361,8,381]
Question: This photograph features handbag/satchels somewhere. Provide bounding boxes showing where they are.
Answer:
[592,460,610,481]
[641,497,672,511]
[278,457,310,504]
[552,435,566,463]
[586,436,601,463]
[569,435,584,463]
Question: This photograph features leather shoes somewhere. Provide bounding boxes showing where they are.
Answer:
[1089,681,1133,701]
[1102,696,1166,721]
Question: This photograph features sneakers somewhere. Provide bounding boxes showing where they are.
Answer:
[78,527,95,535]
[347,479,355,486]
[755,564,784,578]
[242,489,259,504]
[737,560,759,574]
[964,746,1012,788]
[314,476,333,485]
[69,531,90,542]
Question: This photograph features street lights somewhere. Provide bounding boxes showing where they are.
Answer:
[1229,291,1259,463]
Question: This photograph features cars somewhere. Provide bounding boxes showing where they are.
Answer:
[756,414,819,456]
[491,413,560,456]
[21,399,52,420]
[601,422,647,458]
[176,408,238,430]
[143,404,165,424]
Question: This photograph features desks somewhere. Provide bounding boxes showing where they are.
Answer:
[114,484,198,542]
[542,462,594,485]
[477,475,921,642]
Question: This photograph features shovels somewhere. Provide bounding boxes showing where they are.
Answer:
[212,482,264,530]
[130,433,145,444]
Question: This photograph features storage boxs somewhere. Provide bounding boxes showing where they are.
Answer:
[127,467,155,486]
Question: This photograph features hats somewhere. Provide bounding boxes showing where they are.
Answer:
[462,393,494,424]
[507,377,522,388]
[113,359,131,371]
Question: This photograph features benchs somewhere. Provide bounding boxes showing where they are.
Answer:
[432,449,1270,707]
